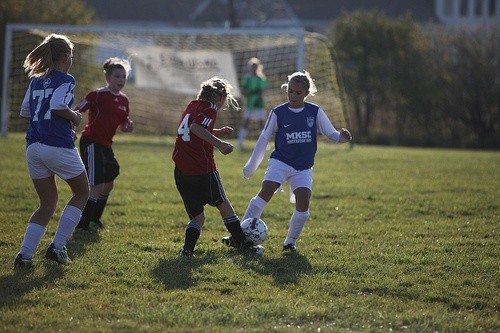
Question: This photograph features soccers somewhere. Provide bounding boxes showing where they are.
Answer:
[239,217,269,246]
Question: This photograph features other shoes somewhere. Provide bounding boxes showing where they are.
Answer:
[180,247,196,258]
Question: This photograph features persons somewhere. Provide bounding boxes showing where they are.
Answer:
[171,77,265,257]
[71,57,135,232]
[238,57,268,150]
[14,33,91,269]
[222,72,353,255]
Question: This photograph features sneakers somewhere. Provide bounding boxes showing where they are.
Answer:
[13,253,34,271]
[43,242,74,265]
[282,242,297,256]
[222,236,237,248]
